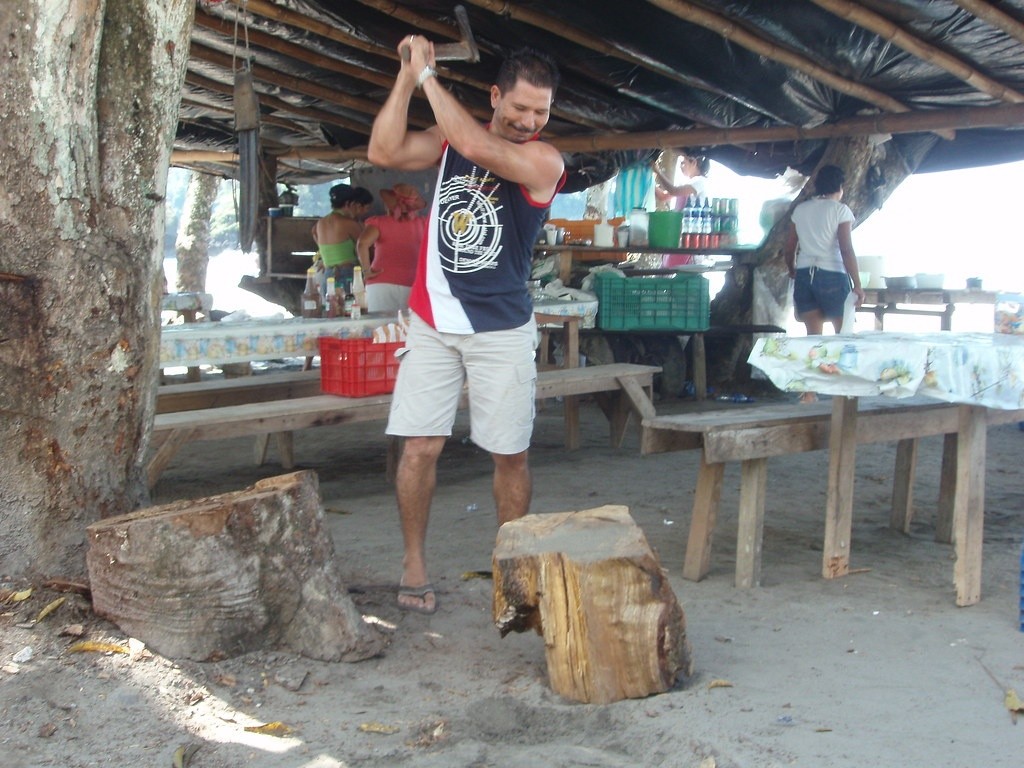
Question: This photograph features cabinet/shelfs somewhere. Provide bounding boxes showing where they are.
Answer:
[262,216,321,283]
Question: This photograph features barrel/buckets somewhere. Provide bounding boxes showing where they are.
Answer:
[647,212,683,249]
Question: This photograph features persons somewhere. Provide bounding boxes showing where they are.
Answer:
[302,182,428,372]
[368,34,567,616]
[653,156,710,278]
[786,165,865,404]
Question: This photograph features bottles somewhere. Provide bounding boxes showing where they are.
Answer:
[302,268,322,318]
[629,206,649,249]
[350,304,360,318]
[325,277,337,318]
[679,195,739,249]
[715,392,754,402]
[352,265,368,315]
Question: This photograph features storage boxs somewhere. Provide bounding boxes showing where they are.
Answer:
[317,336,407,399]
[588,270,710,331]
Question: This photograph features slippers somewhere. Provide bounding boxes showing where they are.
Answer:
[396,584,436,614]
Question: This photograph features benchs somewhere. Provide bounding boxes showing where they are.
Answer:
[144,361,664,494]
[637,394,1024,593]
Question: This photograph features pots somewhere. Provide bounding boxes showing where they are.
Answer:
[880,276,916,290]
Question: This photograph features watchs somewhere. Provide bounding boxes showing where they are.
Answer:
[416,64,438,89]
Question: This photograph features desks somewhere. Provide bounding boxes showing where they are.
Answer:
[532,226,776,392]
[852,287,1024,331]
[157,292,213,387]
[158,285,600,451]
[759,332,1024,608]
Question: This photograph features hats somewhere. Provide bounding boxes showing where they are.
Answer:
[380,182,426,221]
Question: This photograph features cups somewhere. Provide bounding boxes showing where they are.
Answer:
[546,230,558,246]
[555,227,565,245]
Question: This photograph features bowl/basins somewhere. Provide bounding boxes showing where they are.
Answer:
[915,273,945,289]
[279,204,293,217]
[268,207,282,217]
